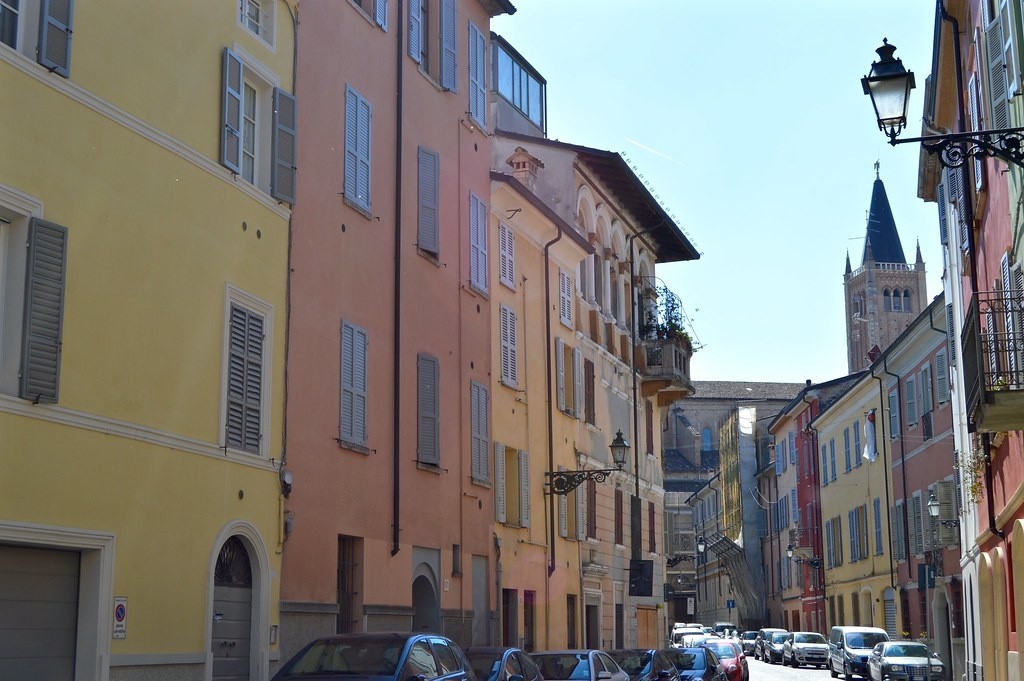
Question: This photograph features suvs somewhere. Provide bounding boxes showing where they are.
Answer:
[755,628,787,661]
[782,632,830,670]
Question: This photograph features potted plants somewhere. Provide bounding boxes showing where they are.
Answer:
[648,311,697,354]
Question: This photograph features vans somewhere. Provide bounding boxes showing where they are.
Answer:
[828,625,891,681]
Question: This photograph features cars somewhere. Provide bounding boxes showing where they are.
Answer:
[524,649,631,681]
[269,631,478,681]
[658,647,729,681]
[705,640,749,681]
[458,645,546,681]
[762,632,790,663]
[700,643,746,681]
[604,649,682,681]
[865,640,947,681]
[670,621,759,655]
